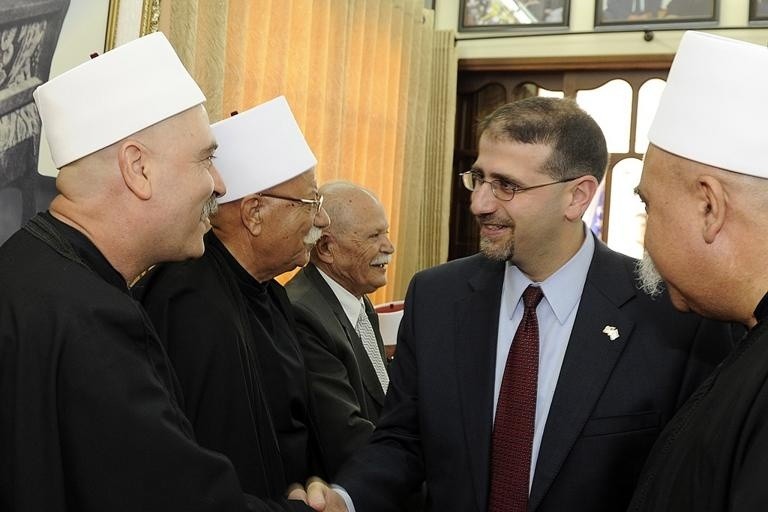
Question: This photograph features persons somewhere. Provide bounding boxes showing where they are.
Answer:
[282,182,396,487]
[0,30,317,512]
[128,94,335,503]
[624,28,767,510]
[287,96,745,512]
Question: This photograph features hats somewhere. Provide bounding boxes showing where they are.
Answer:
[646,28,768,179]
[32,30,209,171]
[208,93,319,206]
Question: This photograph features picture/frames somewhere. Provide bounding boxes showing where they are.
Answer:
[747,0,768,29]
[592,0,721,29]
[458,0,571,33]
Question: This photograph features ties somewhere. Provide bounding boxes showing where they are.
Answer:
[355,302,391,397]
[487,283,545,512]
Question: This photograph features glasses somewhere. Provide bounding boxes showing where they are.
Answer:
[256,192,324,214]
[457,170,586,202]
